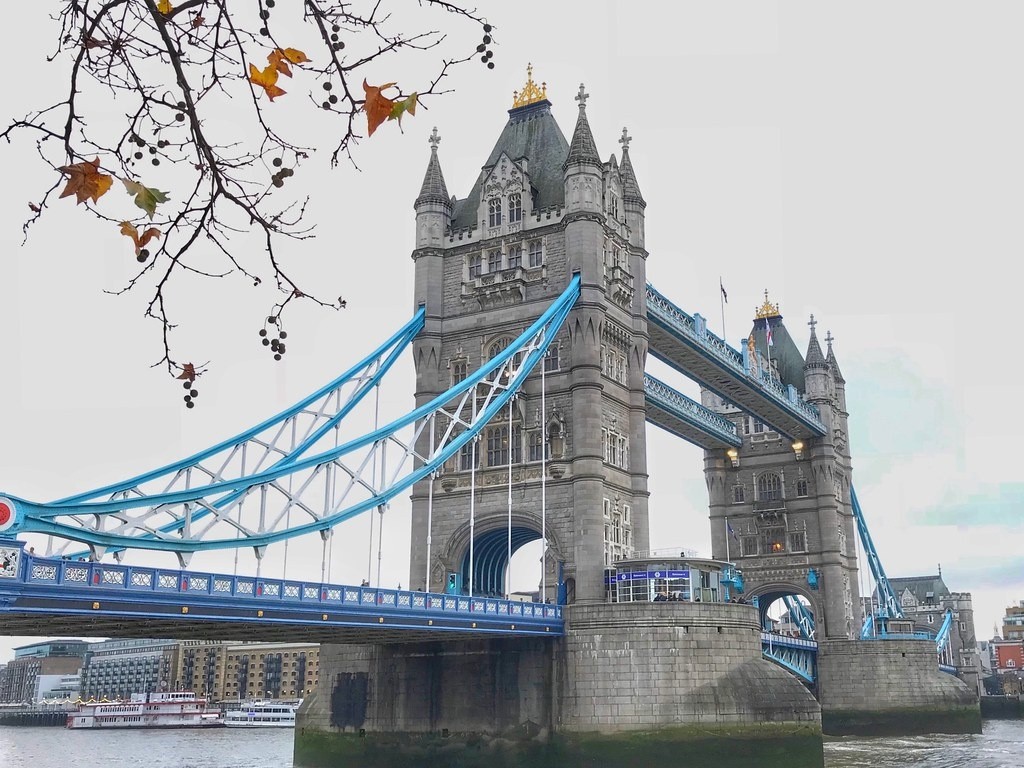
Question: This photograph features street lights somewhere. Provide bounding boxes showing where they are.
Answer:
[0,694,112,712]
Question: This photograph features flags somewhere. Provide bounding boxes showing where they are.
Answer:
[728,523,734,537]
[721,285,727,303]
[766,319,773,346]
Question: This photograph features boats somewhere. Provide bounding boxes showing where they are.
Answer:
[224,697,305,727]
[65,679,225,728]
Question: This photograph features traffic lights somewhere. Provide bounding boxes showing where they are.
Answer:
[449,573,456,589]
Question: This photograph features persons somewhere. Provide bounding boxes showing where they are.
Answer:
[732,596,748,604]
[78,557,86,572]
[654,591,684,602]
[88,555,93,562]
[62,556,71,564]
[29,547,36,557]
